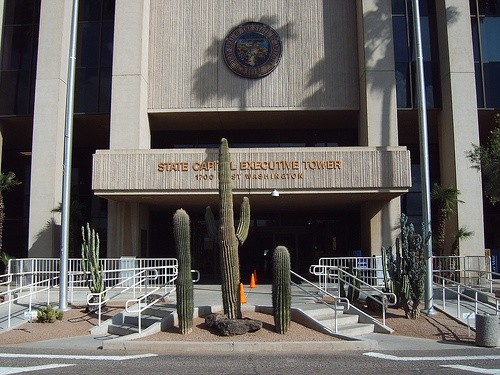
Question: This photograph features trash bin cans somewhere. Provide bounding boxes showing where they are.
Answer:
[117,254,140,288]
[373,255,394,287]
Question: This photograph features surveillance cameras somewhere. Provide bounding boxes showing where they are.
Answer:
[270,188,279,201]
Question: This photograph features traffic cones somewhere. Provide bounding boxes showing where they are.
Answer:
[254,270,258,281]
[240,283,247,304]
[246,273,258,288]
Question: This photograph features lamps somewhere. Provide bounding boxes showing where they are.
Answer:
[271,188,280,198]
